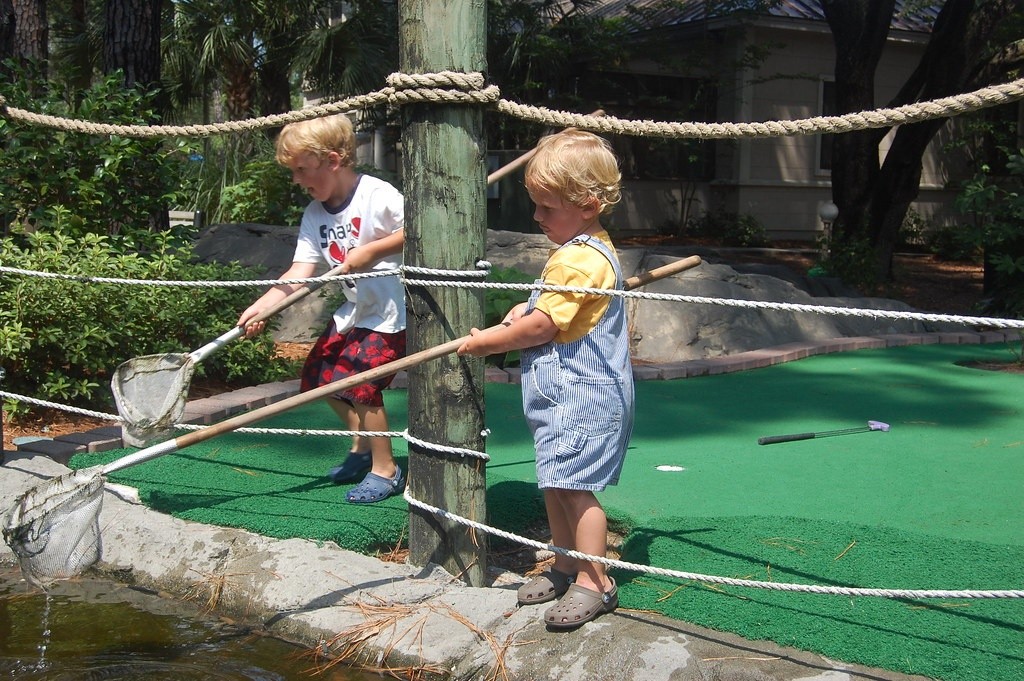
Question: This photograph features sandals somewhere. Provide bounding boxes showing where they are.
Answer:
[345,464,405,503]
[544,575,618,628]
[517,566,579,605]
[330,450,372,482]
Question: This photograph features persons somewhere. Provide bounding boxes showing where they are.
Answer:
[458,129,636,628]
[237,114,404,504]
